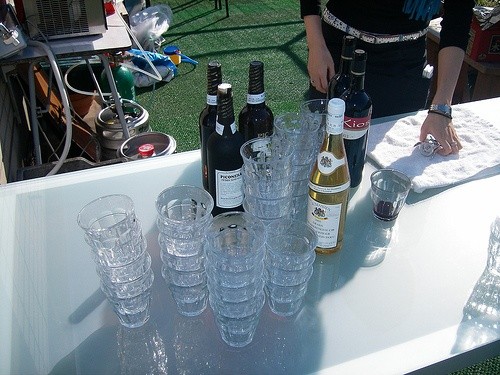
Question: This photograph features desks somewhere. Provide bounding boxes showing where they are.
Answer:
[5,11,133,164]
[1,95,500,375]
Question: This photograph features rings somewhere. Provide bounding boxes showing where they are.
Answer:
[311,80,314,84]
[450,141,457,147]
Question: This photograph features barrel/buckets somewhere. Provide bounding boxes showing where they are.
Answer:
[62,60,176,161]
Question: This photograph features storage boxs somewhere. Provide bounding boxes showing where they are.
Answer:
[466,16,500,61]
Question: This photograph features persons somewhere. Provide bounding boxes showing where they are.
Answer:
[299,0,477,157]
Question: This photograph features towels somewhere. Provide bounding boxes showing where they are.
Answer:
[366,104,500,193]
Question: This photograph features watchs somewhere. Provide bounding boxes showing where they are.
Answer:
[428,103,453,118]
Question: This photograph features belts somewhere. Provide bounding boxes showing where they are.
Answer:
[322,6,430,44]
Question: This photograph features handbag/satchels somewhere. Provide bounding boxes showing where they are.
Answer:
[124,48,181,88]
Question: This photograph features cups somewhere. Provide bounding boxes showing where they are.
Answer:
[370,169,411,220]
[487,214,500,278]
[77,98,330,347]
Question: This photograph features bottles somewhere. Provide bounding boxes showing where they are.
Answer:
[340,48,374,185]
[198,61,222,192]
[326,34,358,105]
[308,99,351,255]
[206,83,246,221]
[239,61,274,171]
